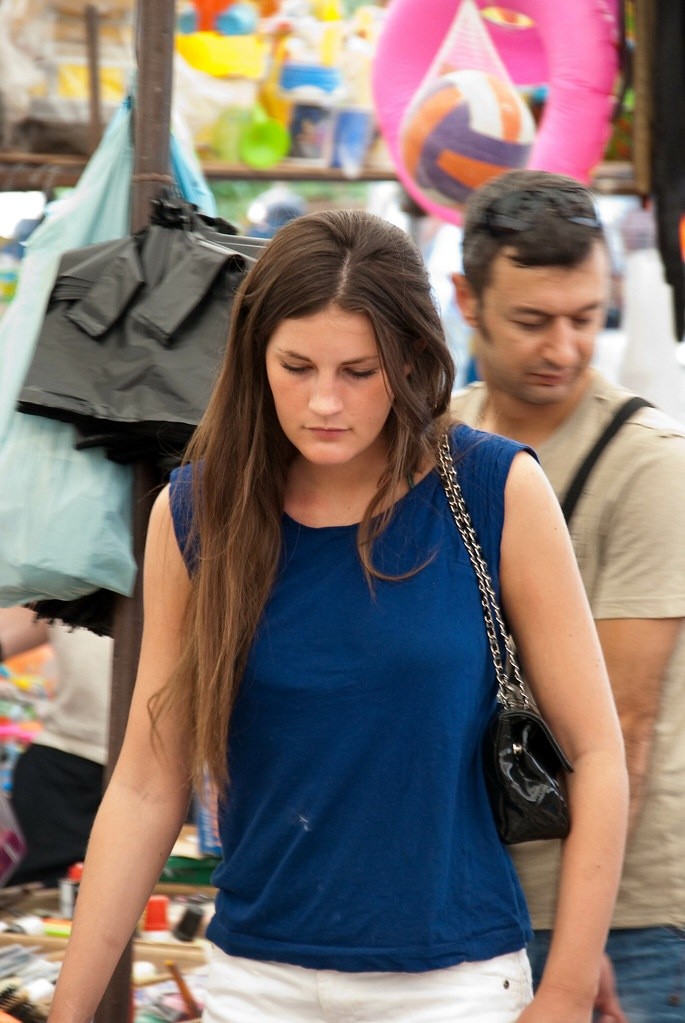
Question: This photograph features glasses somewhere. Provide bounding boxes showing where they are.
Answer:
[466,191,606,233]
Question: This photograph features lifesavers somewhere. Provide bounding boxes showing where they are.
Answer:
[367,2,625,231]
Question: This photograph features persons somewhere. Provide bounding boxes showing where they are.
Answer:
[48,212,634,1023]
[443,172,684,1023]
[0,602,115,891]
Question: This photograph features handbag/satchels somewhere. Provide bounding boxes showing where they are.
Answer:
[432,432,576,846]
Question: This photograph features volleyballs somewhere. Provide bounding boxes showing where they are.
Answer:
[397,69,539,215]
